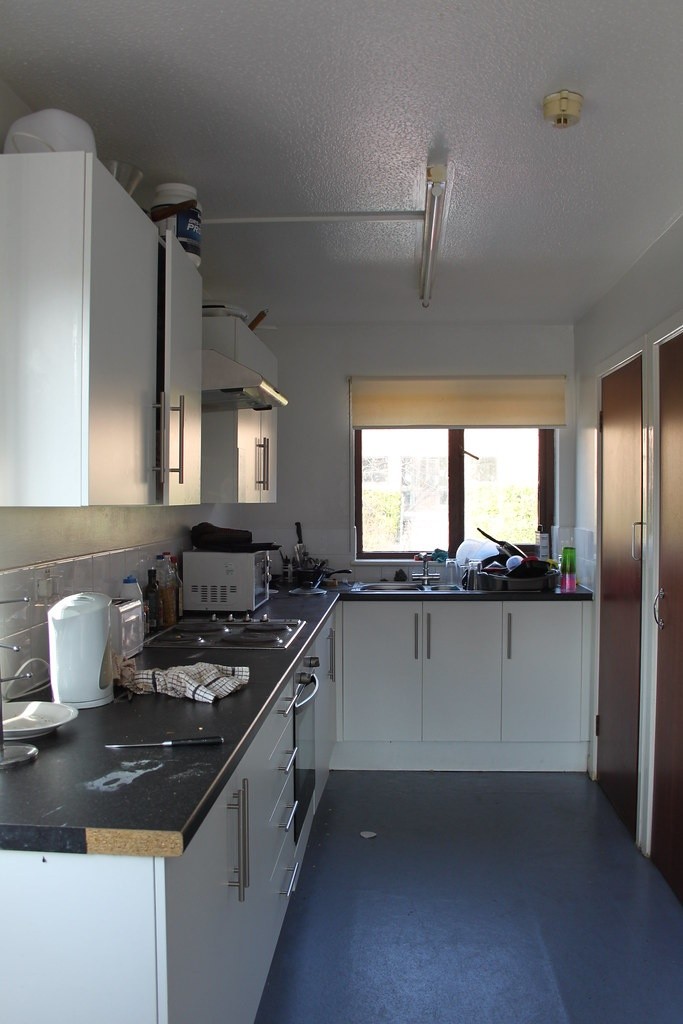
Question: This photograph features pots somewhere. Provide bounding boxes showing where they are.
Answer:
[292,568,352,579]
[477,528,527,560]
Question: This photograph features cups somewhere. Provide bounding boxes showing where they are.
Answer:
[561,547,577,573]
[446,559,457,584]
[562,573,576,592]
[469,559,482,590]
[460,566,470,590]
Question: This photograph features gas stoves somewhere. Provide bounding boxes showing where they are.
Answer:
[143,613,307,650]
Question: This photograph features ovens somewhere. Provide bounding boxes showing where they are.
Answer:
[294,641,320,845]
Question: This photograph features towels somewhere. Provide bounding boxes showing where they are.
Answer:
[111,650,251,704]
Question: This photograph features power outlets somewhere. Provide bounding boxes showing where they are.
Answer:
[32,564,63,601]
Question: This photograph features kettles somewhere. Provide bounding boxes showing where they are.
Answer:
[47,592,114,709]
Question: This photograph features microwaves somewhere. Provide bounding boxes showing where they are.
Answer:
[182,550,272,612]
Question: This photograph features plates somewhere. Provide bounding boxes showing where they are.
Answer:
[2,701,78,740]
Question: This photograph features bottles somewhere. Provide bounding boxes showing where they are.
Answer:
[155,552,178,627]
[143,569,160,637]
[283,555,292,582]
[151,183,203,268]
[120,576,144,640]
[557,555,562,587]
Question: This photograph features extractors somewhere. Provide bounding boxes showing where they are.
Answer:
[201,349,288,411]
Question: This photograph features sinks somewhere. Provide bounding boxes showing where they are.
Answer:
[350,581,426,593]
[431,585,462,592]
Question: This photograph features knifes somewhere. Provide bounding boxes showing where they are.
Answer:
[295,522,304,563]
[104,737,224,748]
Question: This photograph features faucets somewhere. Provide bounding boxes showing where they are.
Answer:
[412,551,440,587]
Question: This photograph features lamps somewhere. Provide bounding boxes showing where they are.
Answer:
[419,158,446,312]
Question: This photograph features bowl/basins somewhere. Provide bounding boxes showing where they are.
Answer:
[483,569,507,575]
[102,159,144,196]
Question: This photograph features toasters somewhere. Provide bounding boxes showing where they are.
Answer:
[111,598,143,660]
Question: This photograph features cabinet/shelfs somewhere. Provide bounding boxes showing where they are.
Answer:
[503,599,590,772]
[0,688,301,1024]
[304,591,340,803]
[232,317,282,506]
[346,598,503,773]
[0,153,201,510]
